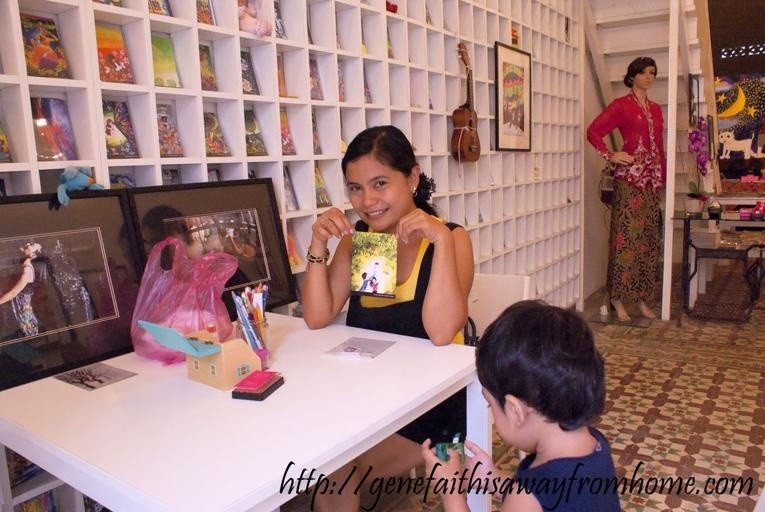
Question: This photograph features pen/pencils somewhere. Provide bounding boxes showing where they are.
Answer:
[231,283,269,363]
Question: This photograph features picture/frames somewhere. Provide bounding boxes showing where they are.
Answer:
[128,177,298,327]
[495,41,531,151]
[693,1,765,199]
[0,189,143,392]
[688,74,699,127]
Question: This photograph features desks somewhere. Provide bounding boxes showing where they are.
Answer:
[0,307,495,512]
[671,210,765,314]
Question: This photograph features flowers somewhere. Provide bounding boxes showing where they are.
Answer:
[686,116,712,203]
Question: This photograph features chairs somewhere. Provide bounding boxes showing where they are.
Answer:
[462,273,532,462]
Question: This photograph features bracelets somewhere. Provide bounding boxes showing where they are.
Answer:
[305,244,330,263]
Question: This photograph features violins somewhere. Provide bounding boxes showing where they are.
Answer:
[452,42,480,162]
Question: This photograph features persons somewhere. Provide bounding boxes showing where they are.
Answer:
[586,56,666,321]
[140,205,250,292]
[220,226,244,255]
[299,124,475,511]
[422,299,622,511]
[96,226,140,335]
[0,241,47,337]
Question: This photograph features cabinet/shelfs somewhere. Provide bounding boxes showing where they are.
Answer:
[0,0,586,512]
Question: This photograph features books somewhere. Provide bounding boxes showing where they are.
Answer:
[84,494,108,511]
[232,376,285,401]
[0,1,393,214]
[285,218,303,270]
[2,445,44,489]
[14,487,60,512]
[235,369,282,392]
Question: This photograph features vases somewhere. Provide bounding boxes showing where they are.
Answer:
[701,200,710,220]
